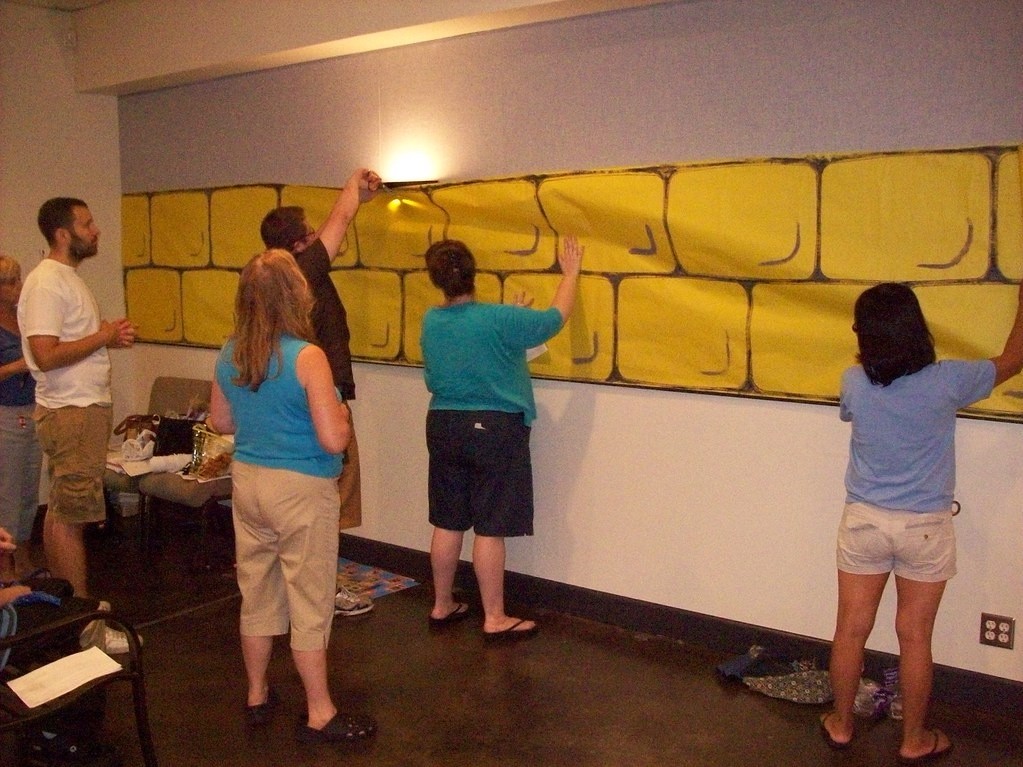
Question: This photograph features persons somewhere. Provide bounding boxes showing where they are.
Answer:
[419,235,585,643]
[0,527,115,767]
[15,197,144,654]
[817,279,1023,767]
[210,246,377,747]
[261,168,383,618]
[0,252,52,588]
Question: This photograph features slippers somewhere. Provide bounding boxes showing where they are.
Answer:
[245,681,276,718]
[482,618,538,641]
[817,709,854,749]
[429,600,470,625]
[895,720,956,765]
[299,714,374,741]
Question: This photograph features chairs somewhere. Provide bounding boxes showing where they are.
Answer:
[0,608,158,767]
[140,437,235,561]
[103,375,211,557]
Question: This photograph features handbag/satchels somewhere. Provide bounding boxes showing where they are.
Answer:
[113,413,161,447]
[188,422,233,481]
[152,415,205,456]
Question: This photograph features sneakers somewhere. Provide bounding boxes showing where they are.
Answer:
[104,625,144,655]
[97,600,111,612]
[334,582,374,616]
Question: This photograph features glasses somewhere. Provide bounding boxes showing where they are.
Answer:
[305,228,315,236]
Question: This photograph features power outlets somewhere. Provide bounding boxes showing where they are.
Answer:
[979,612,1016,650]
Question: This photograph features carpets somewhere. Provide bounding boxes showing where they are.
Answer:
[335,557,419,602]
